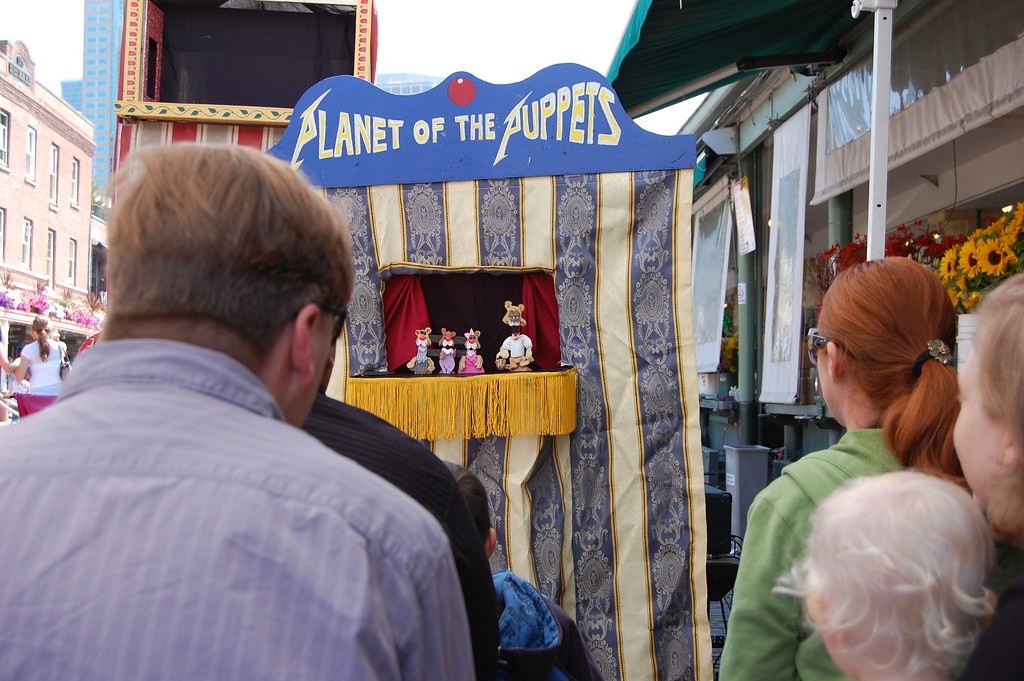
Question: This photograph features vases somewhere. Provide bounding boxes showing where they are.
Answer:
[718,371,738,401]
[958,313,977,371]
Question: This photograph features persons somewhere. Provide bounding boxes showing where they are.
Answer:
[0,315,67,422]
[786,471,992,681]
[0,142,474,681]
[296,307,498,680]
[952,273,1024,681]
[718,259,973,681]
[441,459,604,681]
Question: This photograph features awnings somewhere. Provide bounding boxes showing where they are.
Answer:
[605,0,875,119]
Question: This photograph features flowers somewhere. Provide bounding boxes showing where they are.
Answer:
[721,331,738,372]
[0,271,103,330]
[939,202,1024,313]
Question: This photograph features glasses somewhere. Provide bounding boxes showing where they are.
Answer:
[805,326,838,366]
[295,296,348,344]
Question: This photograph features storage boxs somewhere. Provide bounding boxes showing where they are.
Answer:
[696,374,717,398]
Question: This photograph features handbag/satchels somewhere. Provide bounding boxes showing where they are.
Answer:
[59,346,73,380]
